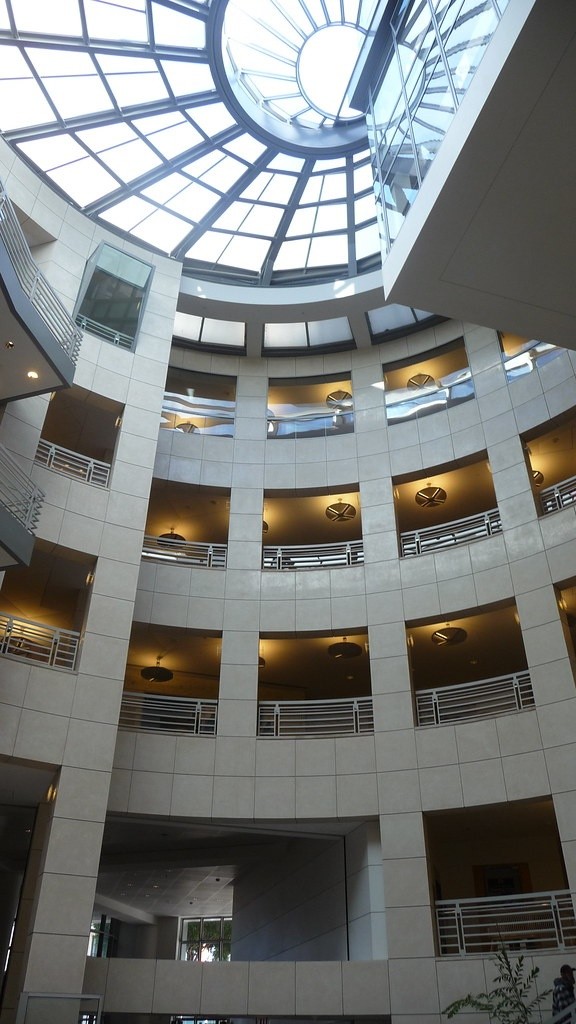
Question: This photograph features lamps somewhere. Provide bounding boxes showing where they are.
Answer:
[431,627,468,648]
[175,423,201,435]
[325,390,353,410]
[327,642,363,660]
[532,470,545,488]
[406,374,435,391]
[325,503,357,522]
[156,533,186,548]
[415,487,447,508]
[140,666,174,683]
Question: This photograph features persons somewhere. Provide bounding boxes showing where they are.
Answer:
[187,944,194,961]
[552,963,576,1024]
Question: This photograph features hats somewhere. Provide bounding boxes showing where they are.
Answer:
[561,965,575,973]
